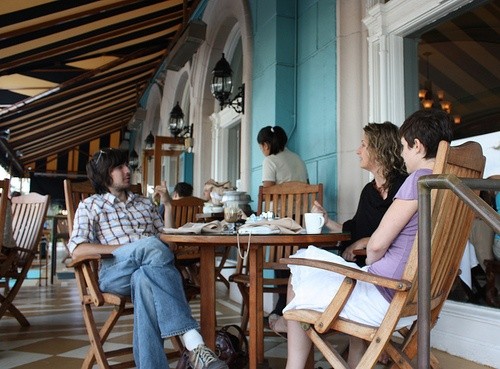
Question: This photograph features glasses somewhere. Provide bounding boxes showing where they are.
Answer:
[94,148,111,164]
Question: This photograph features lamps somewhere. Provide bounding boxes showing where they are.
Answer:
[210,52,245,115]
[145,129,155,149]
[167,101,193,151]
[419,52,460,123]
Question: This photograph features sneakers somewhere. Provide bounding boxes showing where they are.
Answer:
[188,346,229,369]
[268,313,289,342]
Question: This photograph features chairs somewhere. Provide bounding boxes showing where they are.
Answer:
[279,141,486,369]
[229,182,323,349]
[64,180,187,369]
[0,192,51,329]
[164,196,209,300]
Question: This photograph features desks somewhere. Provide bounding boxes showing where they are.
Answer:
[44,215,68,284]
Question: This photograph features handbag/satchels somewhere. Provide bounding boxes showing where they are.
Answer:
[234,216,302,260]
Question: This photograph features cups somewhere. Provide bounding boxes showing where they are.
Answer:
[304,213,325,234]
[224,200,240,222]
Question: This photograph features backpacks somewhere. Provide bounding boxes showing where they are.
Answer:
[211,323,248,369]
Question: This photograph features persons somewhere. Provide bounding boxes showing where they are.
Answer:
[256,124,310,328]
[268,108,455,369]
[158,181,194,221]
[309,120,412,369]
[66,147,229,368]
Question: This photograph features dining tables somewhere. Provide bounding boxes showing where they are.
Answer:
[160,233,356,369]
